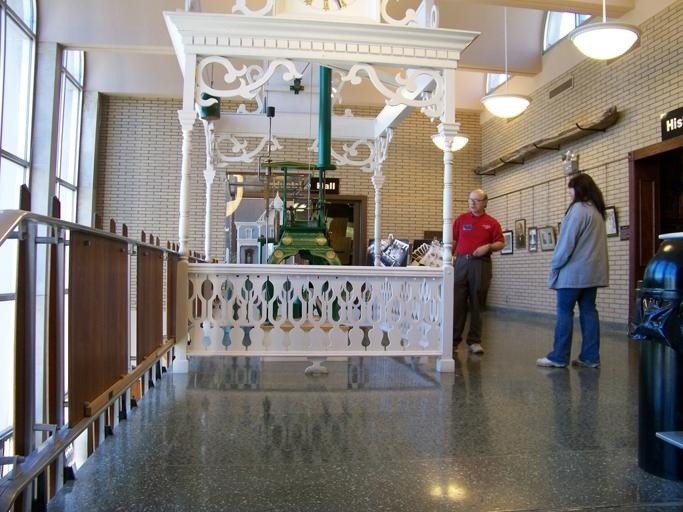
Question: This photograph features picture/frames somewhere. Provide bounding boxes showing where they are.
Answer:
[500,206,619,255]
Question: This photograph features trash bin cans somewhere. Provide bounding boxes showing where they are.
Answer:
[636,233,683,481]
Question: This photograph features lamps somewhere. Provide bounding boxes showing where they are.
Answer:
[480,7,532,119]
[430,134,469,152]
[568,0,640,60]
[198,64,221,120]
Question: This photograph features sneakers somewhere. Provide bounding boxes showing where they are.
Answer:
[572,357,600,368]
[536,357,567,368]
[469,343,483,354]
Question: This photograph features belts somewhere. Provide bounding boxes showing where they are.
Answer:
[464,255,490,263]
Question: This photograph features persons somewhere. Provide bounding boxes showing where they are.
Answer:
[535,174,610,368]
[452,190,505,353]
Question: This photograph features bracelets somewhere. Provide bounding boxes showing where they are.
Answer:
[487,243,492,250]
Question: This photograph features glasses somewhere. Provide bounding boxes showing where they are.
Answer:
[467,199,483,202]
